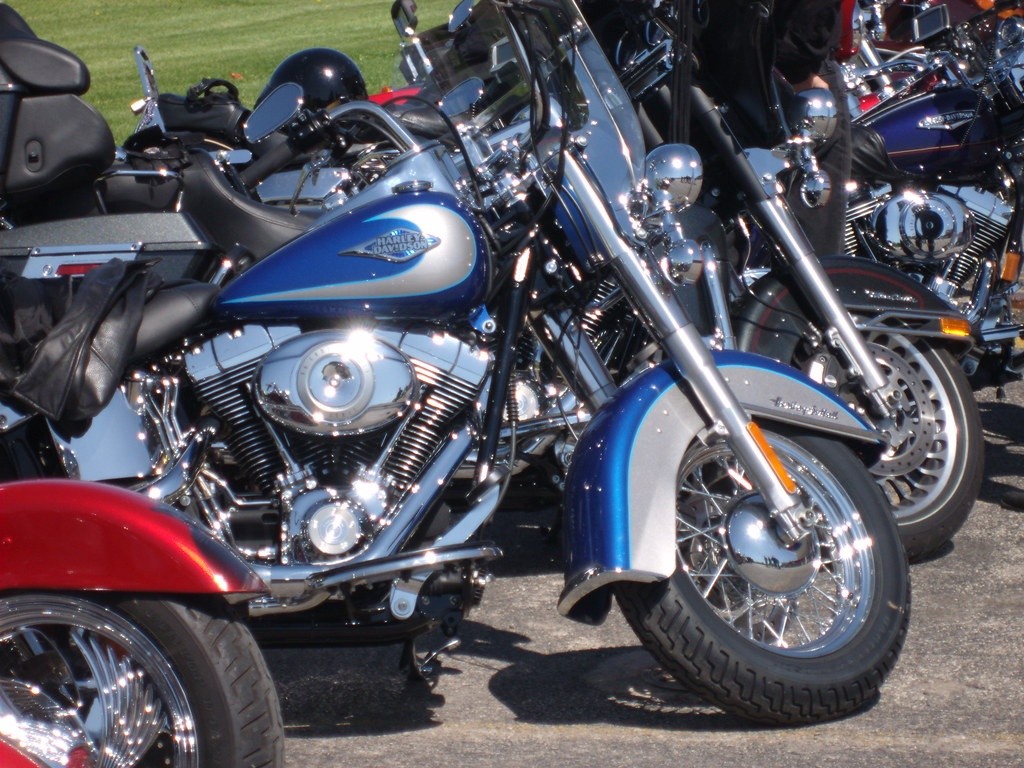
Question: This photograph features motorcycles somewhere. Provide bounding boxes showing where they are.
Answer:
[0,1,909,724]
[400,1,1023,405]
[1,2,286,768]
[131,2,981,559]
